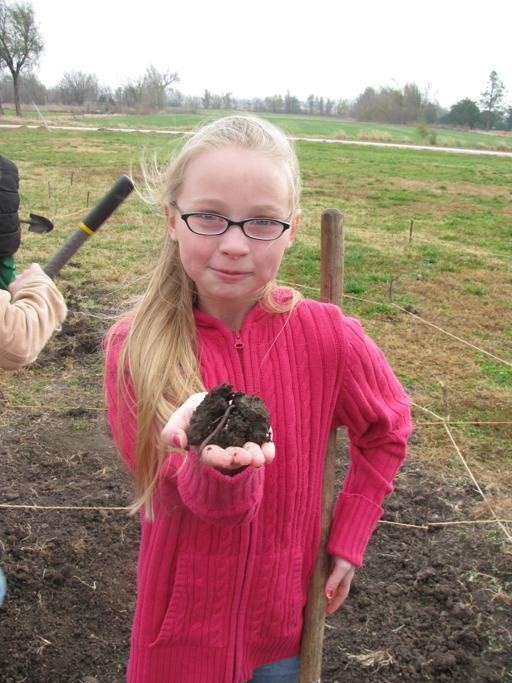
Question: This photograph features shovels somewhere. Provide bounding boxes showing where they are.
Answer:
[18,213,53,234]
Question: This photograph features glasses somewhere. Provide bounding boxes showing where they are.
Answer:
[170,201,290,241]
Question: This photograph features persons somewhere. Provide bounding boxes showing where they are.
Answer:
[0,156,21,290]
[0,262,68,608]
[104,115,413,682]
